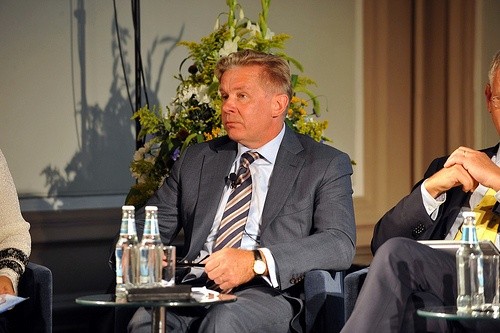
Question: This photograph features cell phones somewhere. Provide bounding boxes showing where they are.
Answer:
[126,286,190,302]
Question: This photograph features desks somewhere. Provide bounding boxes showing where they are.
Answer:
[416,302,500,333]
[75,286,238,332]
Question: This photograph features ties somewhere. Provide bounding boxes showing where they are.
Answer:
[453,188,499,246]
[203,152,262,308]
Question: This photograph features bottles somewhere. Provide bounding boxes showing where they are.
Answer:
[139,206,164,287]
[455,212,484,314]
[115,205,140,303]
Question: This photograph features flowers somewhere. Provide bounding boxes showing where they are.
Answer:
[129,0,333,208]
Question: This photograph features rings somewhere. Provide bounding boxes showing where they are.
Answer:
[463,150,466,156]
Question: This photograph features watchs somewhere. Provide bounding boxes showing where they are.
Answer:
[252,250,266,276]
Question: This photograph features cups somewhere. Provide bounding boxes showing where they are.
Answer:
[145,245,179,288]
[467,255,500,319]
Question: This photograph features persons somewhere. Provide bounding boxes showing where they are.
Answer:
[338,51,500,333]
[0,149,47,333]
[108,50,357,333]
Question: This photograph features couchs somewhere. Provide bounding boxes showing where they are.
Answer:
[0,225,368,333]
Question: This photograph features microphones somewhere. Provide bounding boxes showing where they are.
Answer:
[230,173,236,188]
